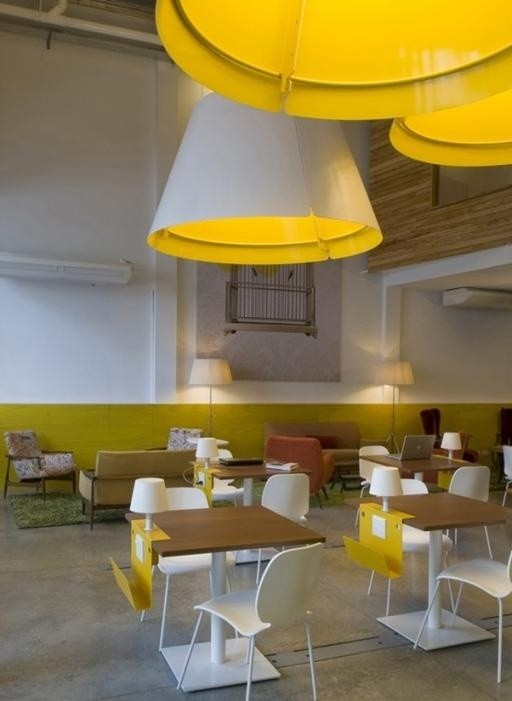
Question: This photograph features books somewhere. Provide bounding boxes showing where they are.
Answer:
[266,460,300,472]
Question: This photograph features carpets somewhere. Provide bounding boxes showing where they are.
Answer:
[10,477,512,530]
[10,457,512,530]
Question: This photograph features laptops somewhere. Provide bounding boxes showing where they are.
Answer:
[218,456,264,466]
[384,433,436,461]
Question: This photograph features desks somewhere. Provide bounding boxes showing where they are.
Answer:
[127,505,326,692]
[346,494,509,652]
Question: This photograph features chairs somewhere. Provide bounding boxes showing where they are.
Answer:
[254,473,310,588]
[3,431,76,501]
[414,540,512,683]
[446,466,492,558]
[140,488,238,650]
[143,427,512,509]
[367,479,455,619]
[497,407,512,482]
[176,540,323,700]
[420,408,479,464]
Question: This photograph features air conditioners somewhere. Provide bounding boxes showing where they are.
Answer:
[0,251,133,286]
[443,287,511,310]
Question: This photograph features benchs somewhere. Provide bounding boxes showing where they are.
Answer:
[78,449,197,531]
[264,421,361,449]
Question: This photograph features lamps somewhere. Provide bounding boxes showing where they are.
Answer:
[130,478,170,530]
[188,359,234,440]
[146,91,384,265]
[389,90,512,167]
[155,0,512,122]
[376,361,413,452]
[368,466,402,512]
